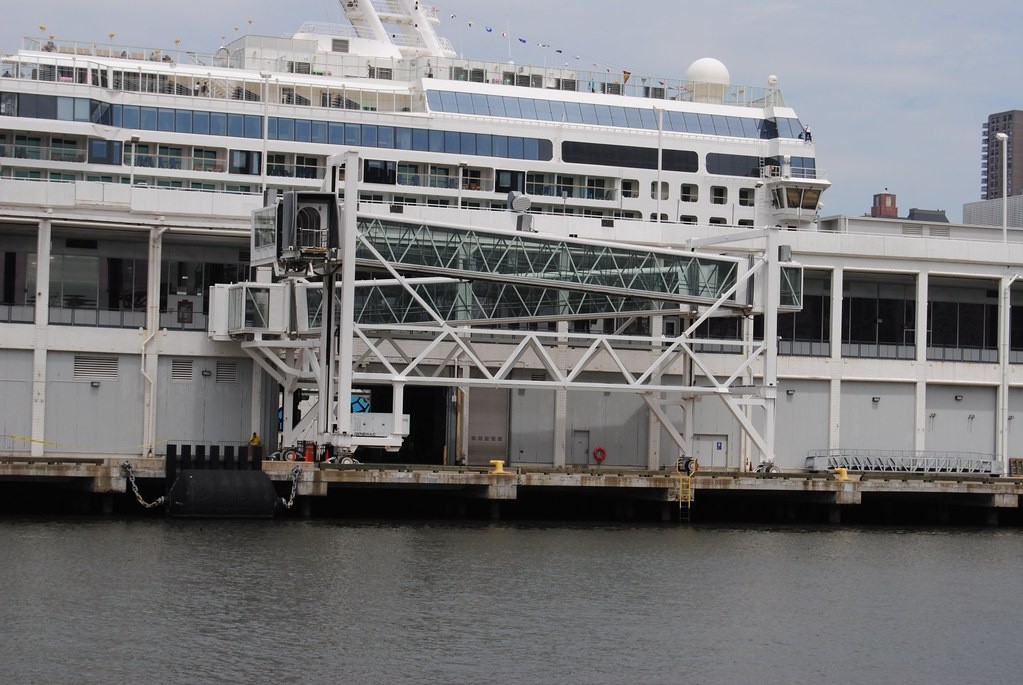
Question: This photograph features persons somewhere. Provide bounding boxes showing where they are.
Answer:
[804,125,812,142]
[248,433,261,446]
[194,81,209,98]
[798,132,804,139]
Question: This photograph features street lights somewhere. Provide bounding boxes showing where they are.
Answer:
[457,161,469,210]
[996,132,1010,243]
[129,134,141,186]
[753,180,765,227]
[259,70,274,196]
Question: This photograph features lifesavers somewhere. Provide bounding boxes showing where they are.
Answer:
[341,457,353,470]
[593,447,607,462]
[284,451,296,461]
